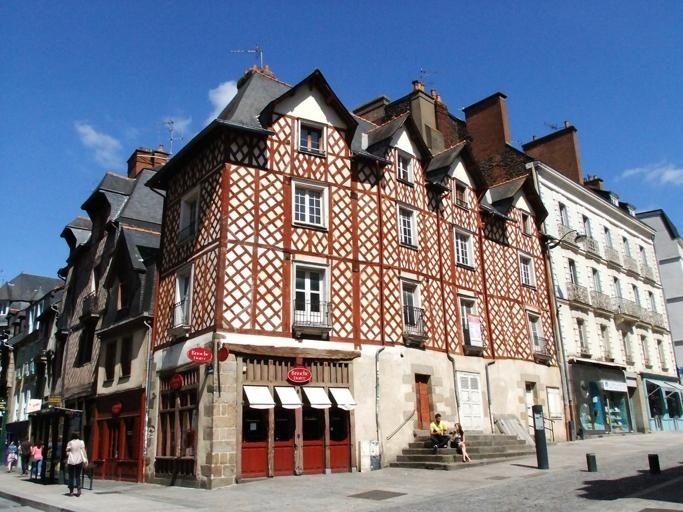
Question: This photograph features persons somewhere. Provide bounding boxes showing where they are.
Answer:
[5,434,63,480]
[451,423,471,462]
[65,433,88,497]
[430,413,451,449]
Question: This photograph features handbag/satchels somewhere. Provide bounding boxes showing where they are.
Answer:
[81,449,88,470]
